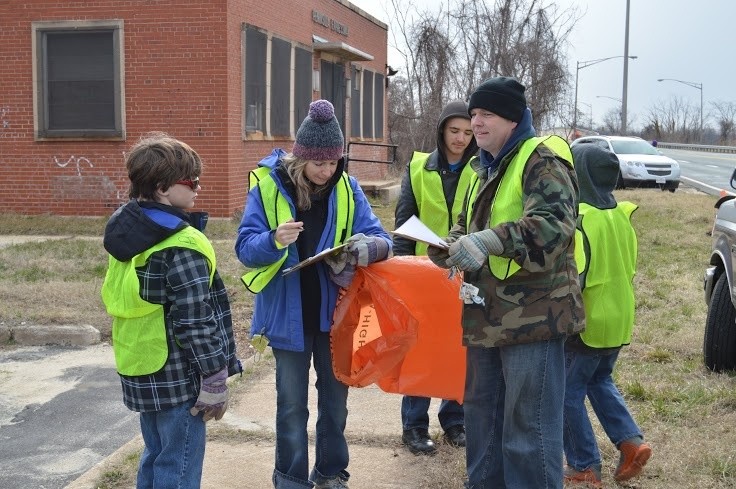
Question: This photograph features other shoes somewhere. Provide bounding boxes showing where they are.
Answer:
[612,436,652,482]
[563,462,602,488]
[314,477,350,489]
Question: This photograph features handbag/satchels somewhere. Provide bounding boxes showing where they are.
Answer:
[330,255,466,405]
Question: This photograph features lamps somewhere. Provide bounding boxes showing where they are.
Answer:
[385,63,398,75]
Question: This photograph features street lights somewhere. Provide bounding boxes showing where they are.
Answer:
[656,78,703,130]
[572,54,639,140]
[596,95,628,136]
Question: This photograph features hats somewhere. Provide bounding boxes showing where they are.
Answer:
[293,100,346,161]
[467,76,527,124]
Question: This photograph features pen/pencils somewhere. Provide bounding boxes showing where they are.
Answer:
[439,242,449,248]
[286,221,304,229]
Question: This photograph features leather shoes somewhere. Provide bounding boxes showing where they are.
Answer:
[442,424,466,448]
[402,427,435,455]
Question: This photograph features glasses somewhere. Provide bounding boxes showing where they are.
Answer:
[175,177,200,191]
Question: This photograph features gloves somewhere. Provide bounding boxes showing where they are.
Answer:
[189,369,229,423]
[427,234,468,270]
[321,234,389,288]
[445,229,505,274]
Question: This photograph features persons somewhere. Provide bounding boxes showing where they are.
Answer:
[562,144,652,487]
[427,76,586,489]
[101,133,243,489]
[392,101,480,454]
[234,100,392,489]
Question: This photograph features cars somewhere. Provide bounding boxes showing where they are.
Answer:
[569,134,681,193]
[701,195,736,373]
